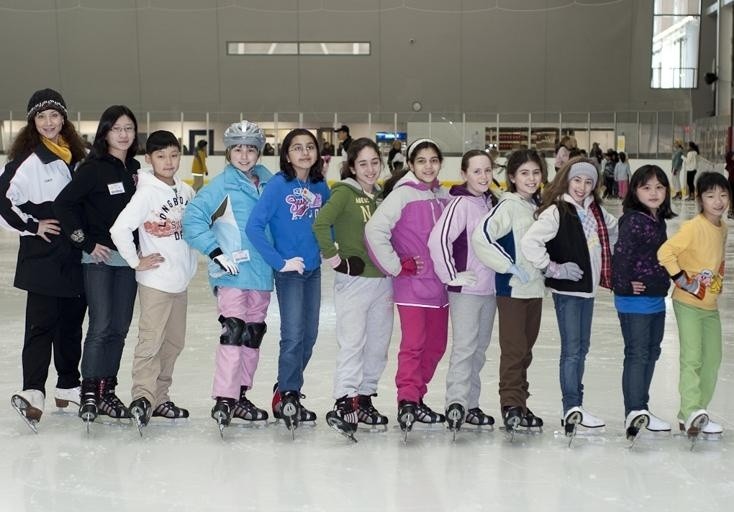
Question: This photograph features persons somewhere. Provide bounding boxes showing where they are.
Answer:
[109,129,196,429]
[520,155,620,437]
[364,139,452,432]
[312,138,397,433]
[83,123,733,219]
[427,149,497,430]
[471,149,546,432]
[245,127,334,429]
[610,165,678,440]
[54,106,141,423]
[0,89,92,421]
[182,120,273,425]
[657,172,731,433]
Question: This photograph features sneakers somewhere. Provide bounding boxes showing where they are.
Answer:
[327,394,388,429]
[627,409,670,441]
[14,379,189,424]
[679,410,725,437]
[398,400,544,429]
[212,385,317,428]
[562,408,604,436]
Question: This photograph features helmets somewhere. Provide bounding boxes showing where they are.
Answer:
[223,119,266,153]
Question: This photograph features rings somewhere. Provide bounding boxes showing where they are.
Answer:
[49,229,51,234]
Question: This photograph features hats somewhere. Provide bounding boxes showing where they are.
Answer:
[27,88,67,124]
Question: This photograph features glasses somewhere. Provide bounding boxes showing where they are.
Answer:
[110,126,133,132]
[287,146,315,154]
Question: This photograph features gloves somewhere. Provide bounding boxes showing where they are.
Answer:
[279,256,306,275]
[328,253,365,275]
[445,271,477,287]
[210,248,239,276]
[673,268,704,300]
[507,265,529,286]
[542,261,583,282]
